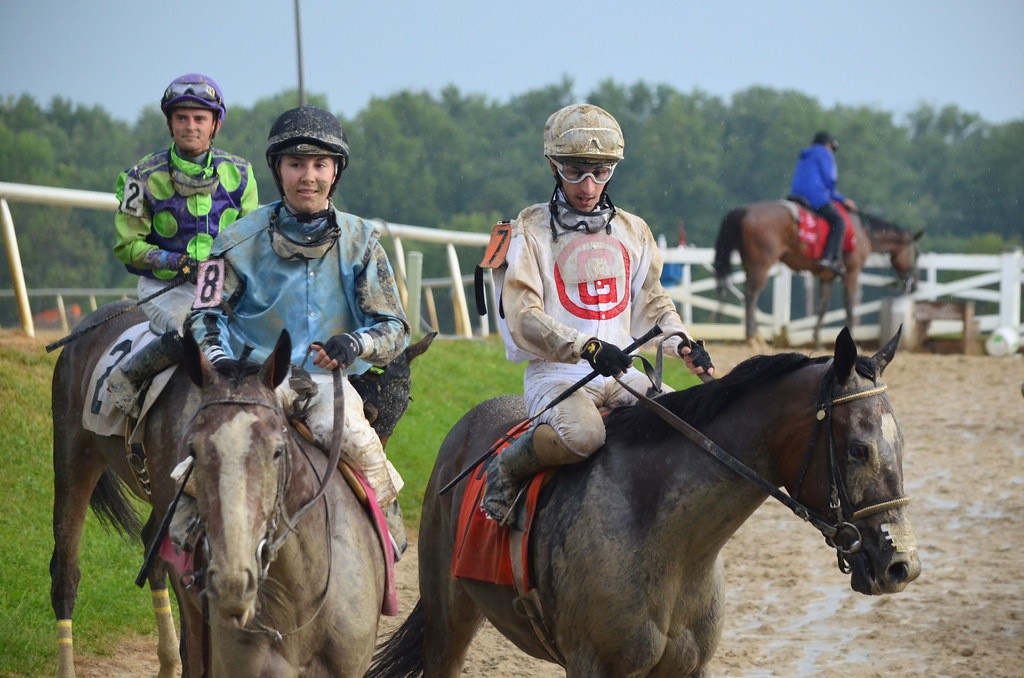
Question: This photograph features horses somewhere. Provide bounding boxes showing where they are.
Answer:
[51,299,439,677]
[143,340,439,678]
[363,324,922,678]
[712,200,928,350]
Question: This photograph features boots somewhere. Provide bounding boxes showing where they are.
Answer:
[483,423,554,525]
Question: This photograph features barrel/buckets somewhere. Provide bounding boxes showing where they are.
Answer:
[985,325,1020,357]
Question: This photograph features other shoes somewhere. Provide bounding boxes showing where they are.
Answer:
[382,497,410,556]
[169,493,197,544]
[816,260,848,279]
[106,366,138,418]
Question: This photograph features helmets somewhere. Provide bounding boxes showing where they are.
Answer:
[544,104,625,161]
[263,104,350,167]
[160,73,226,122]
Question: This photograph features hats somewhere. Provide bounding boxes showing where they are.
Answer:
[812,132,837,152]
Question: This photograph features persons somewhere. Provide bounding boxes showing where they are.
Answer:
[475,104,715,526]
[166,105,410,559]
[791,131,855,273]
[104,72,258,420]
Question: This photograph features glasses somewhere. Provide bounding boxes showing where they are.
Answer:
[557,160,615,184]
[163,83,216,101]
